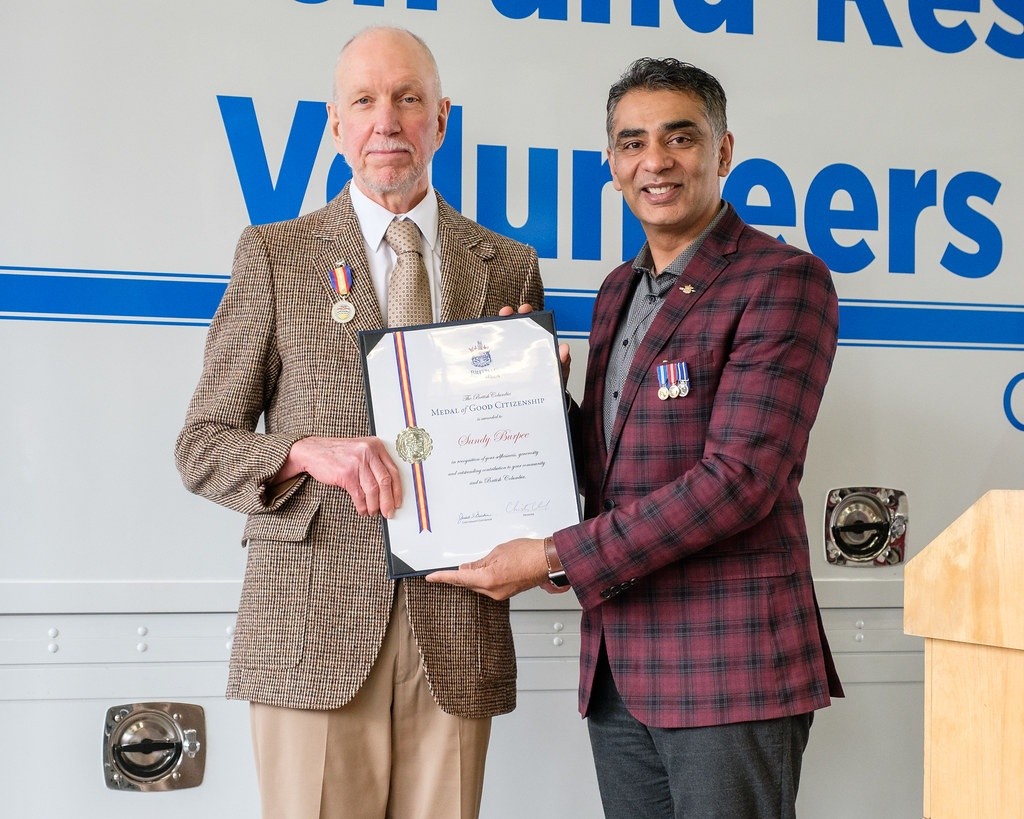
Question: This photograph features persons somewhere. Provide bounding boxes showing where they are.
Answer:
[426,58,845,818]
[176,25,544,817]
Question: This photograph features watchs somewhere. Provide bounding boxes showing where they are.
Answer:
[544,535,571,590]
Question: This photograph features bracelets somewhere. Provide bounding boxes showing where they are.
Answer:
[565,390,572,412]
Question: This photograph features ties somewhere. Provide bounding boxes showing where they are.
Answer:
[383,218,433,329]
